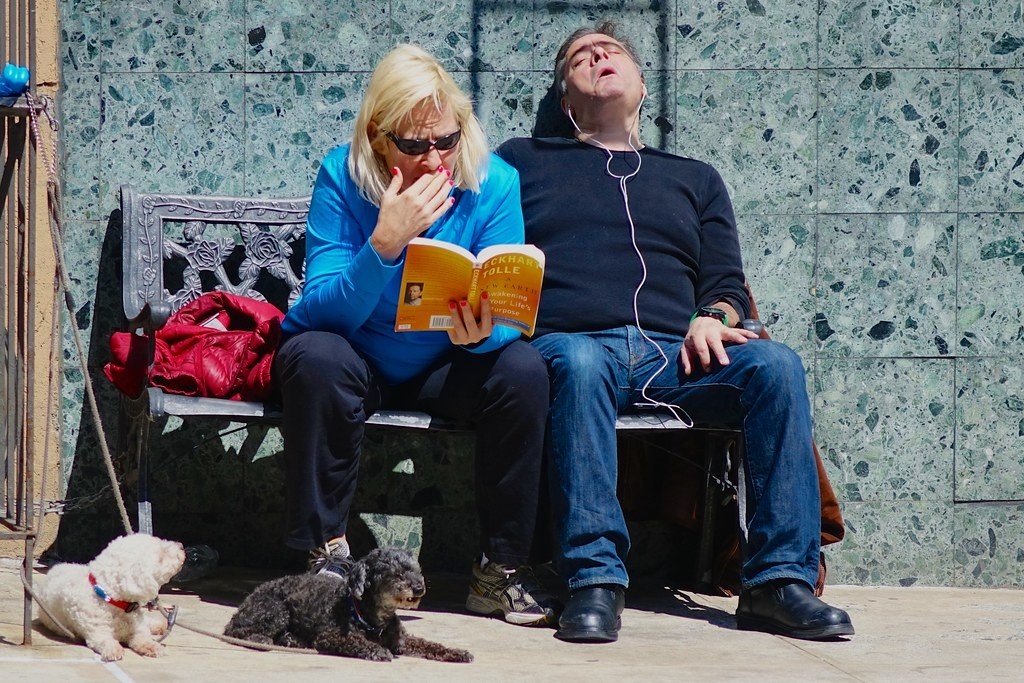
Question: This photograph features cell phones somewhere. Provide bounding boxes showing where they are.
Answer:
[632,403,665,415]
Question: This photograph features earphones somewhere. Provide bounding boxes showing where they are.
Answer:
[568,104,572,111]
[641,83,647,97]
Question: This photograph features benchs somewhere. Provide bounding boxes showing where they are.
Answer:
[115,185,752,593]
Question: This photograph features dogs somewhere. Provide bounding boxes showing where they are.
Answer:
[38,532,187,662]
[221,547,475,664]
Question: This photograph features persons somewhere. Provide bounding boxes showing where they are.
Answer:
[489,20,856,643]
[405,283,422,305]
[274,42,559,627]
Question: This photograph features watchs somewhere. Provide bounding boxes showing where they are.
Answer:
[690,306,728,325]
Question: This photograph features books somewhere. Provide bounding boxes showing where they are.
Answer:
[395,236,546,338]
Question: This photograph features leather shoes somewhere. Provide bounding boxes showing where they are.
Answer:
[554,584,625,641]
[735,579,855,638]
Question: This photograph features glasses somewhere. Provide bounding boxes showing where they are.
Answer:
[383,129,461,155]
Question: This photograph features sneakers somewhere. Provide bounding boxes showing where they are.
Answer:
[465,562,554,627]
[309,538,355,579]
